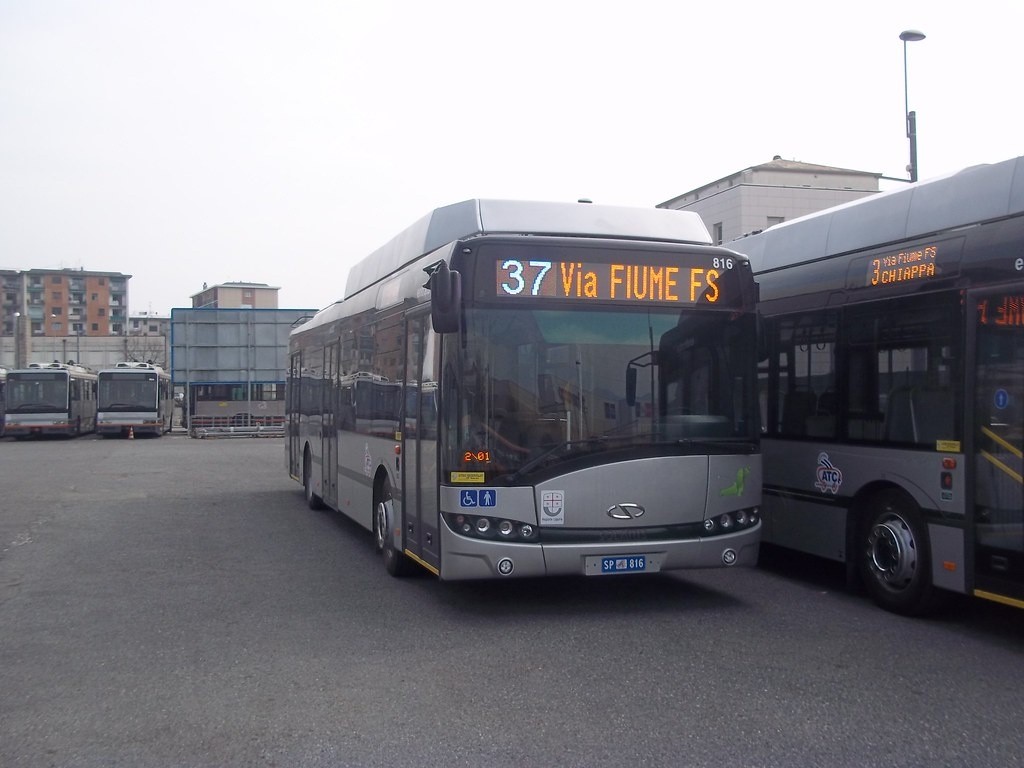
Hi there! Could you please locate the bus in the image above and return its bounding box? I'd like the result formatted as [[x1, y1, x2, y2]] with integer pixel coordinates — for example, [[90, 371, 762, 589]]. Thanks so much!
[[282, 197, 766, 589], [5, 360, 96, 437], [179, 380, 288, 430], [722, 155, 1024, 620], [97, 362, 174, 438]]
